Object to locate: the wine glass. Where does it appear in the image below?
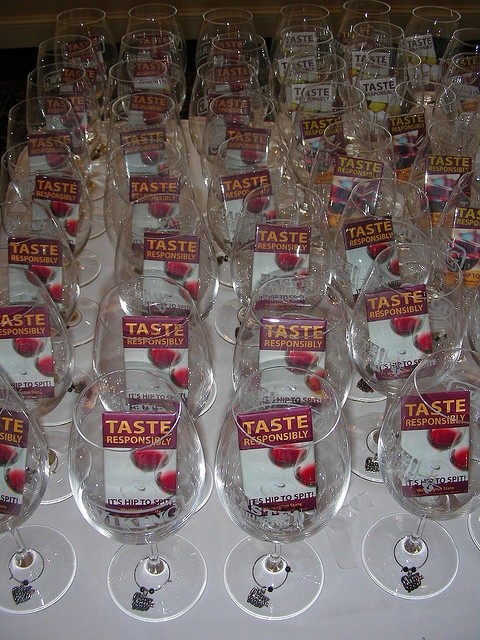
[102,57,180,130]
[101,141,199,253]
[26,61,109,200]
[204,32,276,106]
[337,21,406,106]
[188,59,262,156]
[270,4,337,61]
[125,1,188,80]
[114,191,217,421]
[398,5,461,114]
[436,26,480,93]
[231,276,352,410]
[68,368,208,623]
[426,46,438,82]
[330,178,437,403]
[205,134,298,288]
[277,52,352,121]
[117,28,188,117]
[107,93,190,182]
[91,274,214,512]
[0,198,101,430]
[5,96,107,241]
[195,5,261,80]
[346,242,465,484]
[213,364,355,621]
[466,285,480,463]
[201,93,283,188]
[1,373,77,617]
[366,89,391,134]
[362,349,480,600]
[35,33,107,167]
[290,81,371,186]
[382,80,460,180]
[0,265,96,505]
[349,48,423,131]
[272,24,336,85]
[414,47,427,82]
[436,51,480,113]
[334,0,392,59]
[214,182,330,348]
[54,6,118,83]
[307,120,398,229]
[0,138,104,346]
